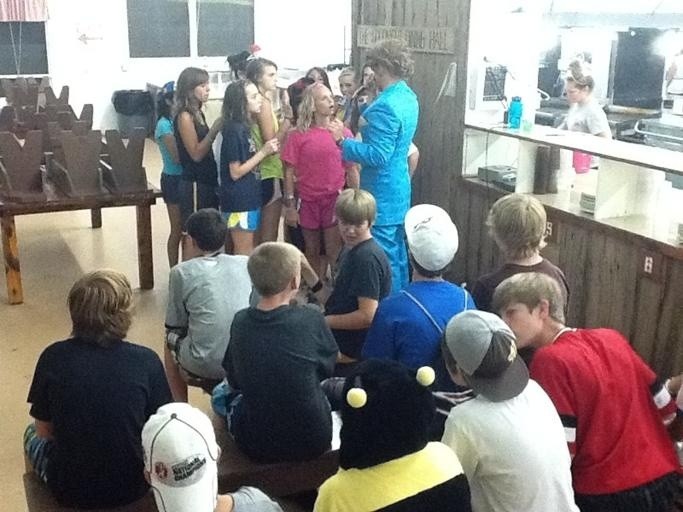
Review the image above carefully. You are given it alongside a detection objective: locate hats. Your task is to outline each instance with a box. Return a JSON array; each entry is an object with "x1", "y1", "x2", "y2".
[
  {"x1": 140, "y1": 401, "x2": 220, "y2": 512},
  {"x1": 444, "y1": 309, "x2": 530, "y2": 404},
  {"x1": 404, "y1": 203, "x2": 460, "y2": 272}
]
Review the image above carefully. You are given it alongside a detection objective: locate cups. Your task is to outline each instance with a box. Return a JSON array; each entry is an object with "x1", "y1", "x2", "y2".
[
  {"x1": 573, "y1": 151, "x2": 591, "y2": 174},
  {"x1": 578, "y1": 190, "x2": 595, "y2": 213}
]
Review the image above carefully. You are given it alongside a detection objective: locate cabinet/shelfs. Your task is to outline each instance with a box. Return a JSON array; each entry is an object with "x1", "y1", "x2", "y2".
[{"x1": 459, "y1": 124, "x2": 682, "y2": 261}]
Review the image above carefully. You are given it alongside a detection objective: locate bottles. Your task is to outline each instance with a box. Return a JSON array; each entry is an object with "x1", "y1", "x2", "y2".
[{"x1": 507, "y1": 97, "x2": 522, "y2": 128}]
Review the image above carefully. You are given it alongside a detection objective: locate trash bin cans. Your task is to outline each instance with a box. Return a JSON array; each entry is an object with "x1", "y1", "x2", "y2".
[{"x1": 111, "y1": 90, "x2": 154, "y2": 138}]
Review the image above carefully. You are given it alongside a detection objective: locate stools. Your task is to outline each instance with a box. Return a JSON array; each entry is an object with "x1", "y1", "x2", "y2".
[{"x1": 0, "y1": 74, "x2": 151, "y2": 199}]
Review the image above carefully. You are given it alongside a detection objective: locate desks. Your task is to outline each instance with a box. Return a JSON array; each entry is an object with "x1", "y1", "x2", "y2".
[{"x1": 0, "y1": 175, "x2": 163, "y2": 306}]
